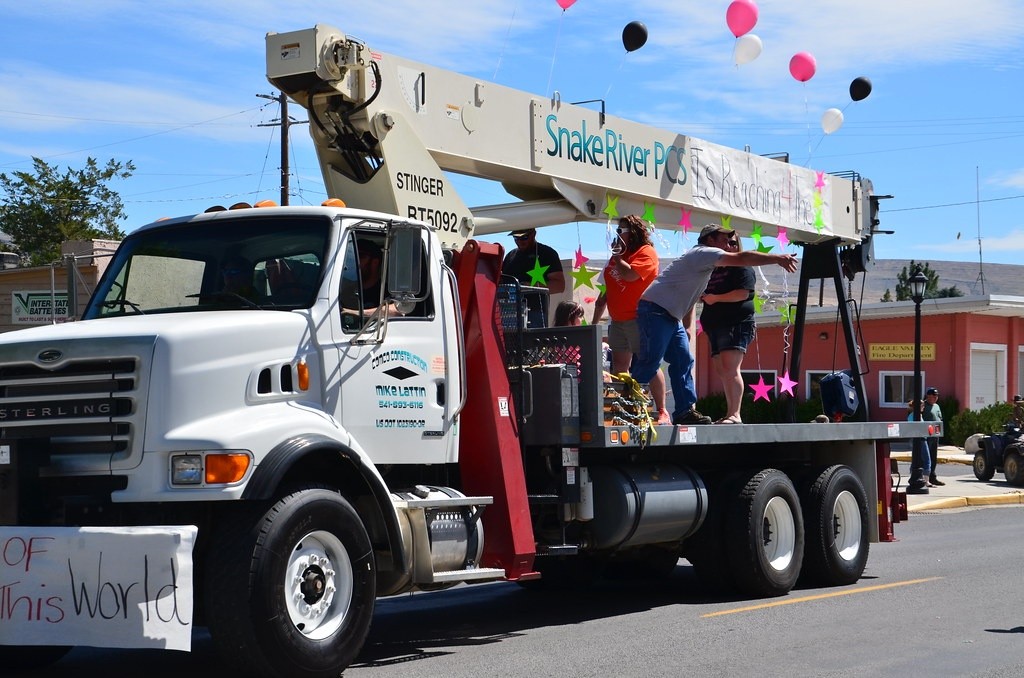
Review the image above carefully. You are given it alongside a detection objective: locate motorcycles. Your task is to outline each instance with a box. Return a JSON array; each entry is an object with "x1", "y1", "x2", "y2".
[{"x1": 972, "y1": 424, "x2": 1024, "y2": 487}]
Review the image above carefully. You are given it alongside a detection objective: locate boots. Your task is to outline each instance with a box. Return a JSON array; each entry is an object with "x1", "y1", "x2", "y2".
[{"x1": 924, "y1": 475, "x2": 932, "y2": 487}]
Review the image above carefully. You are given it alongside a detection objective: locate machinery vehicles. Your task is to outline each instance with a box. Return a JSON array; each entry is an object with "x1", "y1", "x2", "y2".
[{"x1": 0, "y1": 24, "x2": 945, "y2": 678}]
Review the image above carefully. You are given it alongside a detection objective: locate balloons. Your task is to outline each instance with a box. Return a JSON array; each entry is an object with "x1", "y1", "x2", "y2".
[
  {"x1": 735, "y1": 34, "x2": 762, "y2": 64},
  {"x1": 556, "y1": 0, "x2": 576, "y2": 9},
  {"x1": 726, "y1": 0, "x2": 759, "y2": 38},
  {"x1": 850, "y1": 77, "x2": 872, "y2": 100},
  {"x1": 789, "y1": 52, "x2": 816, "y2": 81},
  {"x1": 622, "y1": 21, "x2": 648, "y2": 52},
  {"x1": 823, "y1": 109, "x2": 844, "y2": 134}
]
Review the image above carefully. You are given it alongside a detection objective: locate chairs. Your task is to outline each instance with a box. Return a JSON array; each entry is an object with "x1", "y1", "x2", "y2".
[{"x1": 212, "y1": 257, "x2": 267, "y2": 307}]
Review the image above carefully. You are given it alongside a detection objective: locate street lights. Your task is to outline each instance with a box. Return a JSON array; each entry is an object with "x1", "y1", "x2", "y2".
[{"x1": 904, "y1": 263, "x2": 929, "y2": 495}]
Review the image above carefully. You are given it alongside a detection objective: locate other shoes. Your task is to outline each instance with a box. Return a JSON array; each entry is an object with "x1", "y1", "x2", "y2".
[
  {"x1": 657, "y1": 408, "x2": 670, "y2": 424},
  {"x1": 930, "y1": 479, "x2": 946, "y2": 486}
]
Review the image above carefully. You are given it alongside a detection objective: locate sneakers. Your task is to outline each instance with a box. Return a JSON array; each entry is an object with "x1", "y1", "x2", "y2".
[{"x1": 672, "y1": 409, "x2": 712, "y2": 424}]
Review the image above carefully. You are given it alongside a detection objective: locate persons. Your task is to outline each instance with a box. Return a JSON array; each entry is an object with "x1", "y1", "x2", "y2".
[
  {"x1": 554, "y1": 302, "x2": 584, "y2": 390},
  {"x1": 502, "y1": 228, "x2": 566, "y2": 363},
  {"x1": 632, "y1": 223, "x2": 798, "y2": 424},
  {"x1": 922, "y1": 388, "x2": 945, "y2": 485},
  {"x1": 908, "y1": 400, "x2": 933, "y2": 487},
  {"x1": 699, "y1": 232, "x2": 757, "y2": 423},
  {"x1": 341, "y1": 239, "x2": 405, "y2": 317},
  {"x1": 590, "y1": 215, "x2": 671, "y2": 425}
]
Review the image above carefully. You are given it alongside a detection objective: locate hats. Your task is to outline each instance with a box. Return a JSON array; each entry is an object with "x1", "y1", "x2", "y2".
[
  {"x1": 700, "y1": 223, "x2": 736, "y2": 239},
  {"x1": 927, "y1": 388, "x2": 939, "y2": 395},
  {"x1": 508, "y1": 229, "x2": 532, "y2": 237}
]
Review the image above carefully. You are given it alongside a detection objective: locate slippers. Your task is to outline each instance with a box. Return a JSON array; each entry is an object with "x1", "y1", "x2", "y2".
[{"x1": 715, "y1": 417, "x2": 743, "y2": 424}]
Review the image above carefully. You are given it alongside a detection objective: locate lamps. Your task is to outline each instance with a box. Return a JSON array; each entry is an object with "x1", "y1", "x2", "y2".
[{"x1": 818, "y1": 332, "x2": 828, "y2": 339}]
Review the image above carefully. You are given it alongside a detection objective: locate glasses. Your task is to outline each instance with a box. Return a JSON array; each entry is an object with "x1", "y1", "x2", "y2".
[
  {"x1": 513, "y1": 231, "x2": 532, "y2": 241},
  {"x1": 616, "y1": 227, "x2": 629, "y2": 235},
  {"x1": 728, "y1": 240, "x2": 738, "y2": 246}
]
